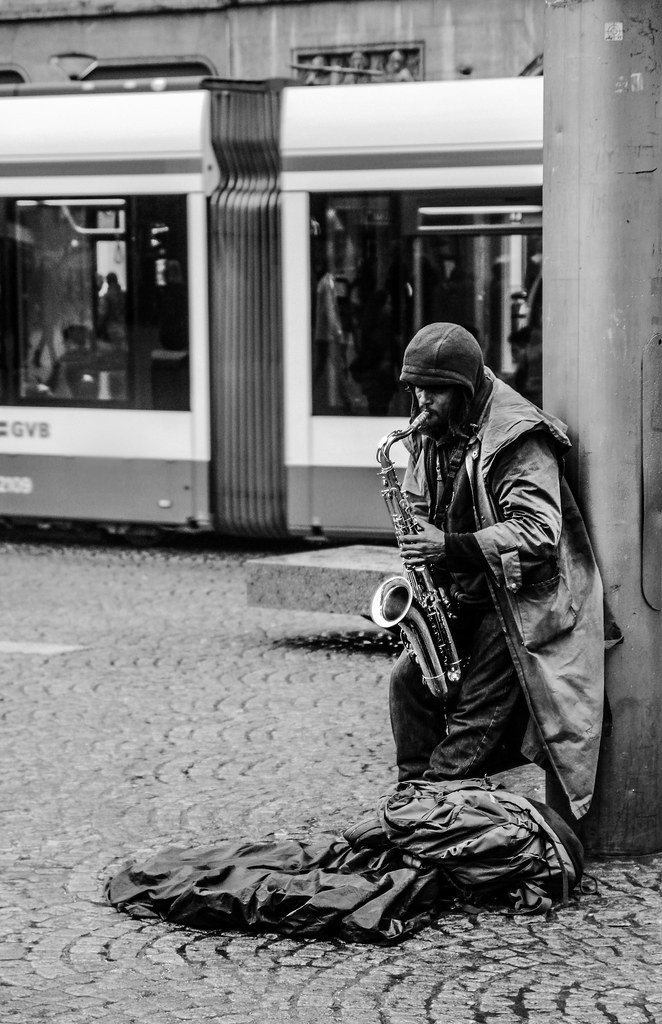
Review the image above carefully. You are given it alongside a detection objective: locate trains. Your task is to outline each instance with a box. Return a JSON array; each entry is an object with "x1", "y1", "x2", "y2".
[{"x1": 0, "y1": 73, "x2": 546, "y2": 547}]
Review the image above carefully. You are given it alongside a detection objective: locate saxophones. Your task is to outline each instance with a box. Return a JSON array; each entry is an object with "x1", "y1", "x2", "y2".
[{"x1": 366, "y1": 412, "x2": 472, "y2": 698}]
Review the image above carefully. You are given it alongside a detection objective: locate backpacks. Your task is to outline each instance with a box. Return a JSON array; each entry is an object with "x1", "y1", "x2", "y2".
[{"x1": 344, "y1": 778, "x2": 571, "y2": 915}]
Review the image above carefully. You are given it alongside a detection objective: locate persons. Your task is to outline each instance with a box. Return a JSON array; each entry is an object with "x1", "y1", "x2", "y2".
[
  {"x1": 44, "y1": 268, "x2": 190, "y2": 396},
  {"x1": 386, "y1": 320, "x2": 608, "y2": 820}
]
